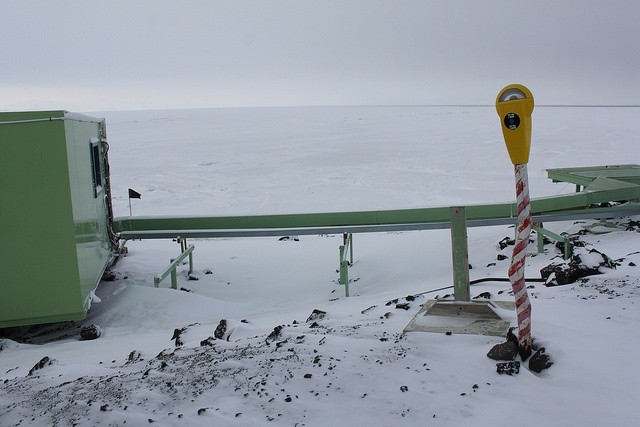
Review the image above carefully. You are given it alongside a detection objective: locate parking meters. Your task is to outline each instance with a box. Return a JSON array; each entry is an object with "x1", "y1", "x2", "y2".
[{"x1": 496, "y1": 83, "x2": 537, "y2": 354}]
